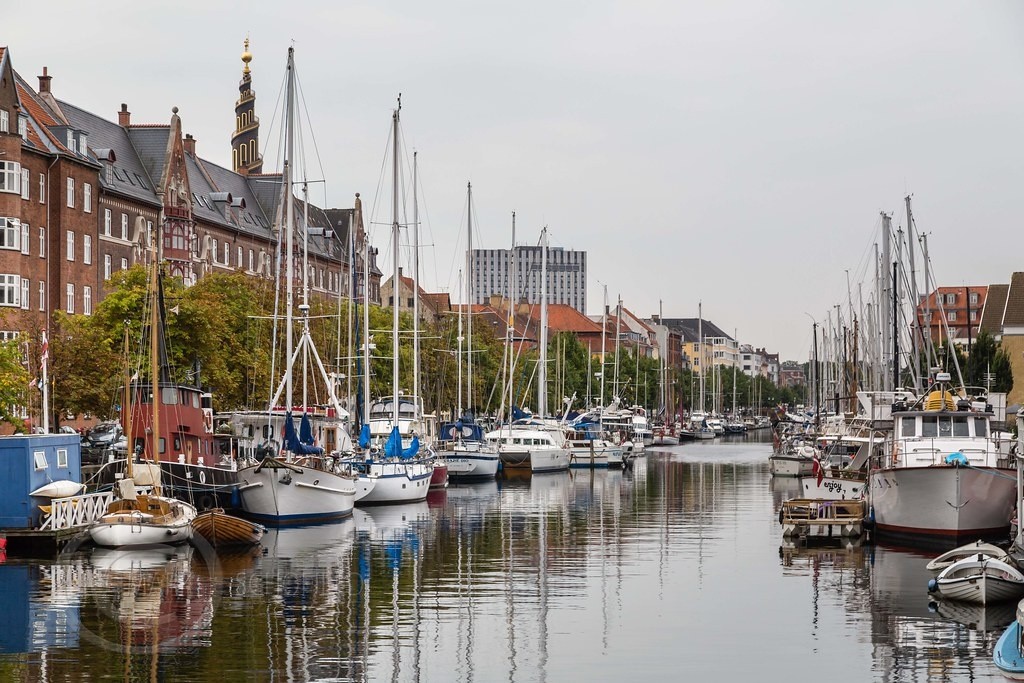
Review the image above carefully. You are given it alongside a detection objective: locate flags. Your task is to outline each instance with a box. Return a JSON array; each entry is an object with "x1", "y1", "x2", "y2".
[
  {"x1": 812, "y1": 453, "x2": 824, "y2": 488},
  {"x1": 772, "y1": 429, "x2": 781, "y2": 452},
  {"x1": 37, "y1": 336, "x2": 49, "y2": 369}
]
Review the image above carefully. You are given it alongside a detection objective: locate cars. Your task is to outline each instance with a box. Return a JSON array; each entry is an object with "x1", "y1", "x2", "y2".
[{"x1": 87, "y1": 423, "x2": 123, "y2": 449}]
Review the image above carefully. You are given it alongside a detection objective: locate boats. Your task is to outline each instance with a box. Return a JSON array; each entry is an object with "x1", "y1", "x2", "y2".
[
  {"x1": 927, "y1": 591, "x2": 1019, "y2": 642},
  {"x1": 992, "y1": 599, "x2": 1024, "y2": 681},
  {"x1": 925, "y1": 539, "x2": 1010, "y2": 578},
  {"x1": 937, "y1": 552, "x2": 1024, "y2": 606}
]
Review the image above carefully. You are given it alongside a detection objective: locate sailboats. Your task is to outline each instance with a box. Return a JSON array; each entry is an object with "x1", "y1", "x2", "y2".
[{"x1": 0, "y1": 39, "x2": 1023, "y2": 552}]
